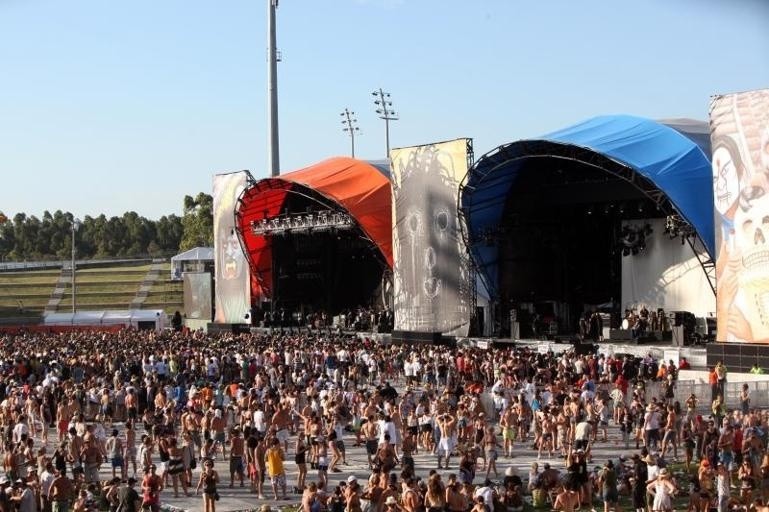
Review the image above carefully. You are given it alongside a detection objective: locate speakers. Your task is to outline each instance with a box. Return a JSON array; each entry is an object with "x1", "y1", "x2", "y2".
[
  {"x1": 672, "y1": 326, "x2": 688, "y2": 347},
  {"x1": 670, "y1": 311, "x2": 682, "y2": 326},
  {"x1": 510, "y1": 322, "x2": 527, "y2": 339},
  {"x1": 510, "y1": 308, "x2": 522, "y2": 322}
]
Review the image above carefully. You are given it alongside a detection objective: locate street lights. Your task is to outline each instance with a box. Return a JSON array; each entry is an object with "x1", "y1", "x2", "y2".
[
  {"x1": 340, "y1": 106, "x2": 362, "y2": 160},
  {"x1": 372, "y1": 87, "x2": 397, "y2": 160}
]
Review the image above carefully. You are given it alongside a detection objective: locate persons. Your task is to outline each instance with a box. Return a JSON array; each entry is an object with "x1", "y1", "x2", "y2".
[{"x1": 0, "y1": 292, "x2": 769, "y2": 510}]
